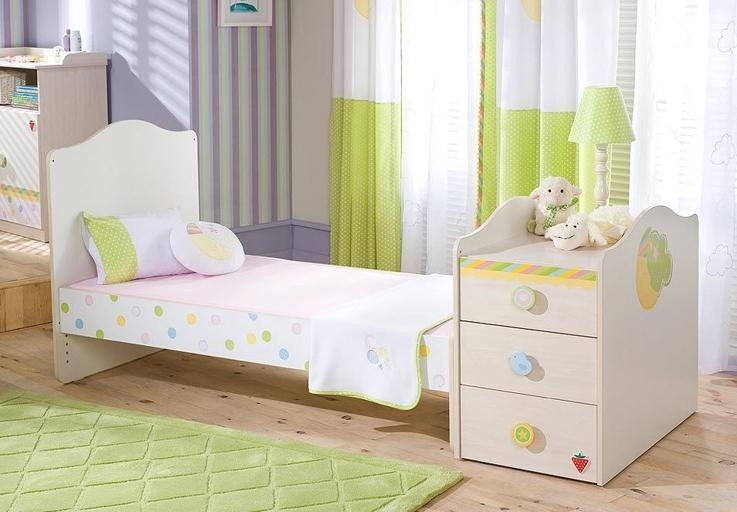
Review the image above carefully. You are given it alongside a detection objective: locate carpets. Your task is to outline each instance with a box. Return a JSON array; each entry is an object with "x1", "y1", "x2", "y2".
[{"x1": 1, "y1": 390, "x2": 468, "y2": 511}]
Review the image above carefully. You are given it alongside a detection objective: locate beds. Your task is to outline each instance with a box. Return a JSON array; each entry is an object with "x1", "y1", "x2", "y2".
[{"x1": 48, "y1": 120, "x2": 450, "y2": 393}]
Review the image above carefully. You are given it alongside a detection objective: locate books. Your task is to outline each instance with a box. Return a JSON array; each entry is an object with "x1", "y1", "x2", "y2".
[{"x1": 0, "y1": 69, "x2": 38, "y2": 112}]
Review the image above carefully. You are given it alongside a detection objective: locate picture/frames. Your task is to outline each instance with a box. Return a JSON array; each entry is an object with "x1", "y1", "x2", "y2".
[{"x1": 216, "y1": 1, "x2": 274, "y2": 27}]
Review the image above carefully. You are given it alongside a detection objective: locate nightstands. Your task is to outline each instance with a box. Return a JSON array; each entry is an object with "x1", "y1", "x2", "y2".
[{"x1": 451, "y1": 198, "x2": 700, "y2": 488}]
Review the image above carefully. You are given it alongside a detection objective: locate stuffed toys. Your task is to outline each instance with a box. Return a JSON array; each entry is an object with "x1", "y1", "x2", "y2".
[
  {"x1": 526, "y1": 176, "x2": 584, "y2": 237},
  {"x1": 541, "y1": 211, "x2": 624, "y2": 252}
]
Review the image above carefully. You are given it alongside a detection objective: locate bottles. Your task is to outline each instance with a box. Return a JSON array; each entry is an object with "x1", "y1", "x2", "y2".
[
  {"x1": 70, "y1": 30, "x2": 81, "y2": 52},
  {"x1": 61, "y1": 28, "x2": 70, "y2": 51}
]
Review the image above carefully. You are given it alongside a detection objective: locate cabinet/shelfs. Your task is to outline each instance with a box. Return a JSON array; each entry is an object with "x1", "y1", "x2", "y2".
[{"x1": 0, "y1": 44, "x2": 111, "y2": 242}]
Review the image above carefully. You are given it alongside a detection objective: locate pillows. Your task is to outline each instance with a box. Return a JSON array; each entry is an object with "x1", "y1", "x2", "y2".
[{"x1": 79, "y1": 207, "x2": 246, "y2": 286}]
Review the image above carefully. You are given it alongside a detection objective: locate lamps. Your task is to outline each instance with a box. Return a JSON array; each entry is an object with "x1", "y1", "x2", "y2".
[{"x1": 566, "y1": 86, "x2": 638, "y2": 210}]
